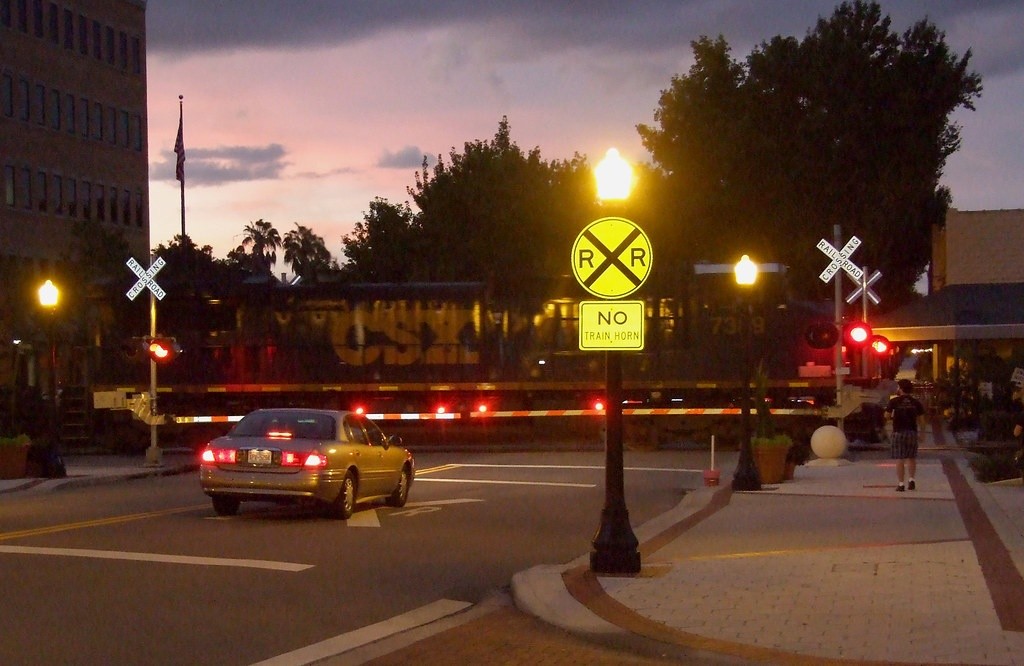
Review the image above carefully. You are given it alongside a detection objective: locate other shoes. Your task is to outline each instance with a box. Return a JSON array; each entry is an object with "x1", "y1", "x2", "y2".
[
  {"x1": 908, "y1": 481, "x2": 915, "y2": 489},
  {"x1": 896, "y1": 485, "x2": 904, "y2": 491}
]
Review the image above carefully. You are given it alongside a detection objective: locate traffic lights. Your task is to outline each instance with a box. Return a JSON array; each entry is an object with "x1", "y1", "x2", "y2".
[
  {"x1": 806, "y1": 322, "x2": 839, "y2": 349},
  {"x1": 868, "y1": 334, "x2": 891, "y2": 357},
  {"x1": 146, "y1": 337, "x2": 181, "y2": 365},
  {"x1": 120, "y1": 337, "x2": 149, "y2": 364},
  {"x1": 843, "y1": 321, "x2": 873, "y2": 348}
]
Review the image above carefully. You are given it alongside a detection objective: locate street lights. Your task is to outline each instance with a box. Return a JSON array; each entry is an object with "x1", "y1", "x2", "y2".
[
  {"x1": 588, "y1": 148, "x2": 640, "y2": 576},
  {"x1": 729, "y1": 255, "x2": 763, "y2": 490},
  {"x1": 38, "y1": 280, "x2": 70, "y2": 479}
]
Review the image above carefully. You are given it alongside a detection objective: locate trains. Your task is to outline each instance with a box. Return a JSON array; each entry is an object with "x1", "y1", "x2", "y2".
[{"x1": 94, "y1": 294, "x2": 850, "y2": 430}]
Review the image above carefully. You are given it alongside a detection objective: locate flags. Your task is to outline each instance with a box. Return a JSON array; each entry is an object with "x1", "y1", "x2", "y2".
[{"x1": 174, "y1": 105, "x2": 187, "y2": 183}]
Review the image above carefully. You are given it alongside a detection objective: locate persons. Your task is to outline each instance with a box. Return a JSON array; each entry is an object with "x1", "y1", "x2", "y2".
[{"x1": 884, "y1": 378, "x2": 927, "y2": 492}]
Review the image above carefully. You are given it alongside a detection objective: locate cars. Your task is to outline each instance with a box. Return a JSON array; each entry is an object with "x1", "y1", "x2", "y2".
[{"x1": 201, "y1": 408, "x2": 417, "y2": 519}]
